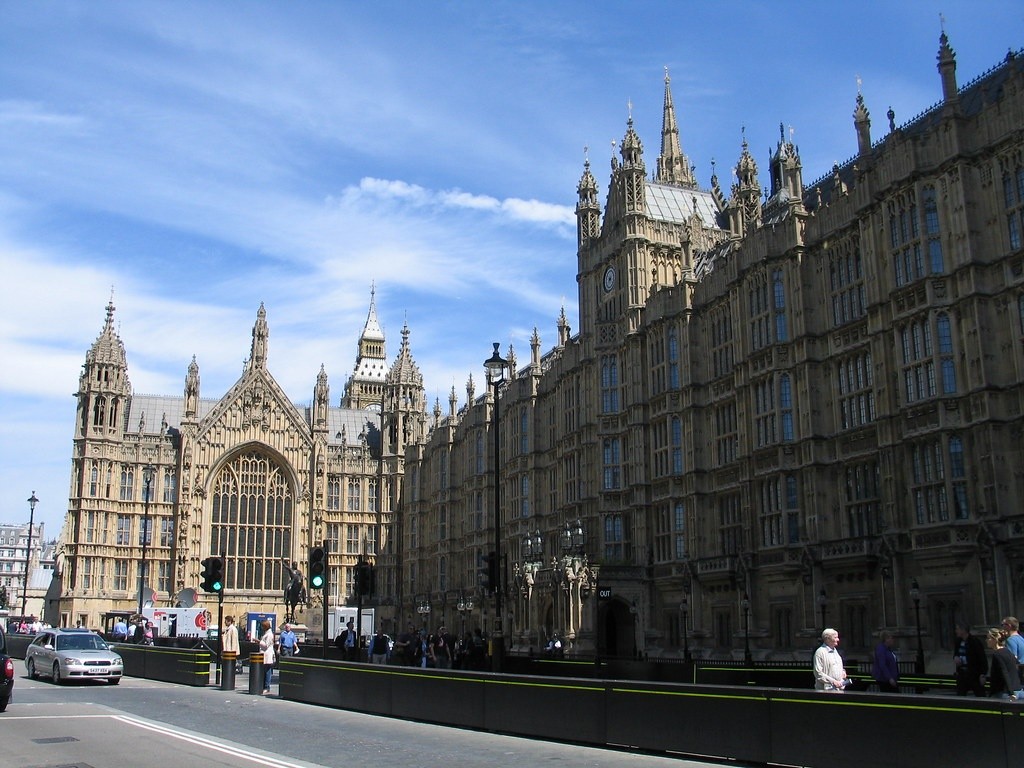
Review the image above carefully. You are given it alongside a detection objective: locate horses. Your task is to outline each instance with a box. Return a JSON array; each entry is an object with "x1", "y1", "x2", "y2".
[{"x1": 284, "y1": 571, "x2": 306, "y2": 623}]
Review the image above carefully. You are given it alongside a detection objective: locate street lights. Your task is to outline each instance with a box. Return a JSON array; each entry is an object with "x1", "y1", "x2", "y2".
[
  {"x1": 378, "y1": 610, "x2": 514, "y2": 650},
  {"x1": 457, "y1": 596, "x2": 473, "y2": 643},
  {"x1": 908, "y1": 581, "x2": 930, "y2": 690},
  {"x1": 17, "y1": 490, "x2": 39, "y2": 634},
  {"x1": 132, "y1": 458, "x2": 158, "y2": 645},
  {"x1": 417, "y1": 600, "x2": 430, "y2": 639},
  {"x1": 817, "y1": 585, "x2": 829, "y2": 631},
  {"x1": 680, "y1": 599, "x2": 688, "y2": 661},
  {"x1": 740, "y1": 596, "x2": 754, "y2": 667},
  {"x1": 481, "y1": 341, "x2": 512, "y2": 673}
]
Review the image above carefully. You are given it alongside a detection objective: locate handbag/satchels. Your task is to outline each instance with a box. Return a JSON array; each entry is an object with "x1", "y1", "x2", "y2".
[{"x1": 236, "y1": 659, "x2": 243, "y2": 675}]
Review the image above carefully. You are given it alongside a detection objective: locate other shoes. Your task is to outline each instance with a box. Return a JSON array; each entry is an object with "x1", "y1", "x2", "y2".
[{"x1": 263, "y1": 689, "x2": 270, "y2": 693}]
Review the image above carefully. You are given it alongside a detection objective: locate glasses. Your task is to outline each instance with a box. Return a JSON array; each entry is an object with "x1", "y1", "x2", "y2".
[
  {"x1": 439, "y1": 627, "x2": 445, "y2": 630},
  {"x1": 408, "y1": 628, "x2": 415, "y2": 630},
  {"x1": 1001, "y1": 624, "x2": 1013, "y2": 626}
]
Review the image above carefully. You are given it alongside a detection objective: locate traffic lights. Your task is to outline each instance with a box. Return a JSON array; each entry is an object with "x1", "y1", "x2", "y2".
[
  {"x1": 199, "y1": 558, "x2": 210, "y2": 592},
  {"x1": 309, "y1": 547, "x2": 326, "y2": 589},
  {"x1": 353, "y1": 563, "x2": 369, "y2": 594},
  {"x1": 481, "y1": 551, "x2": 495, "y2": 591},
  {"x1": 211, "y1": 557, "x2": 224, "y2": 593}
]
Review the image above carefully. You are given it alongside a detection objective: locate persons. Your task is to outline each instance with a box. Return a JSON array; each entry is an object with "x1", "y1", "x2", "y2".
[
  {"x1": 368, "y1": 629, "x2": 391, "y2": 665},
  {"x1": 5, "y1": 613, "x2": 62, "y2": 637},
  {"x1": 280, "y1": 556, "x2": 306, "y2": 606},
  {"x1": 985, "y1": 617, "x2": 1024, "y2": 702},
  {"x1": 71, "y1": 619, "x2": 85, "y2": 630},
  {"x1": 395, "y1": 624, "x2": 493, "y2": 670},
  {"x1": 168, "y1": 614, "x2": 177, "y2": 637},
  {"x1": 870, "y1": 630, "x2": 902, "y2": 693},
  {"x1": 111, "y1": 616, "x2": 154, "y2": 647},
  {"x1": 335, "y1": 621, "x2": 356, "y2": 661},
  {"x1": 223, "y1": 616, "x2": 240, "y2": 656},
  {"x1": 277, "y1": 624, "x2": 300, "y2": 657},
  {"x1": 253, "y1": 620, "x2": 276, "y2": 695},
  {"x1": 544, "y1": 634, "x2": 561, "y2": 652},
  {"x1": 811, "y1": 628, "x2": 853, "y2": 692},
  {"x1": 954, "y1": 619, "x2": 989, "y2": 697}
]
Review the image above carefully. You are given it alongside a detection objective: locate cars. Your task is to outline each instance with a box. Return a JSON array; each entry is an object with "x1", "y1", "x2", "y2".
[
  {"x1": 0, "y1": 624, "x2": 15, "y2": 714},
  {"x1": 24, "y1": 628, "x2": 125, "y2": 686}
]
